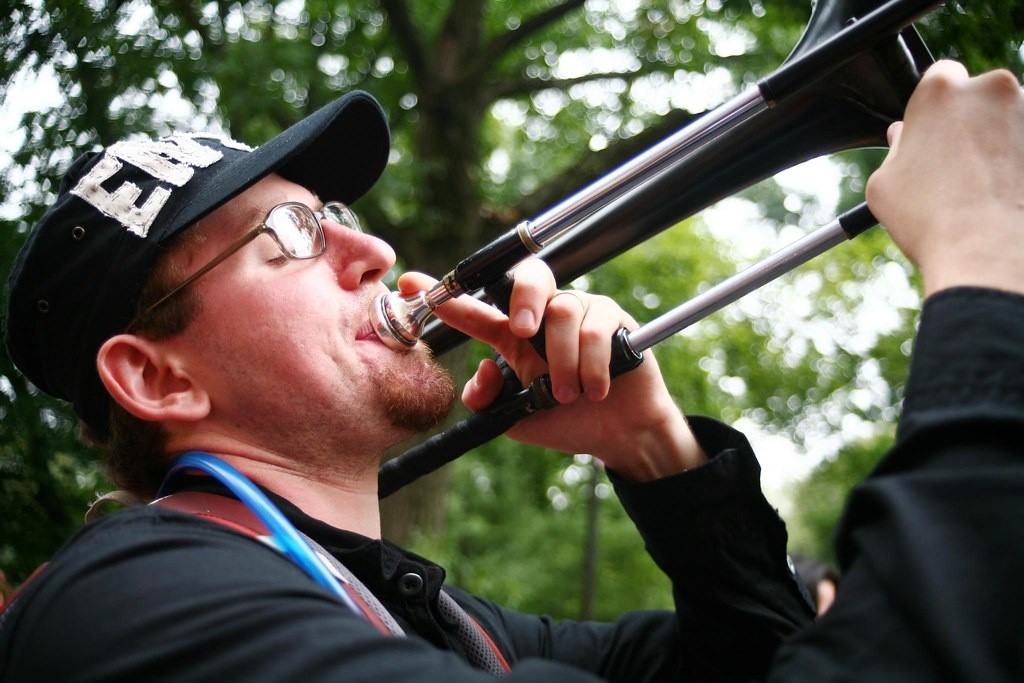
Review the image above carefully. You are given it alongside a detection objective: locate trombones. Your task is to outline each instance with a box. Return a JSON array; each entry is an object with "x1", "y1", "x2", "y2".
[{"x1": 378, "y1": 0, "x2": 1024, "y2": 499}]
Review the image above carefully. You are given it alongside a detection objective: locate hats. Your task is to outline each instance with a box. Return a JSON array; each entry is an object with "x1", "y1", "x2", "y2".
[{"x1": 4, "y1": 89, "x2": 390, "y2": 401}]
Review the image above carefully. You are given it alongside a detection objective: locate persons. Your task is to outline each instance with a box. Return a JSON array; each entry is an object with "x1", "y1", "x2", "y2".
[{"x1": 0, "y1": 59, "x2": 1024, "y2": 683}]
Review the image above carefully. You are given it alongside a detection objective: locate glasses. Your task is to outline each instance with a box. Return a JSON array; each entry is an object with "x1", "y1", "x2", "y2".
[{"x1": 122, "y1": 202, "x2": 364, "y2": 332}]
